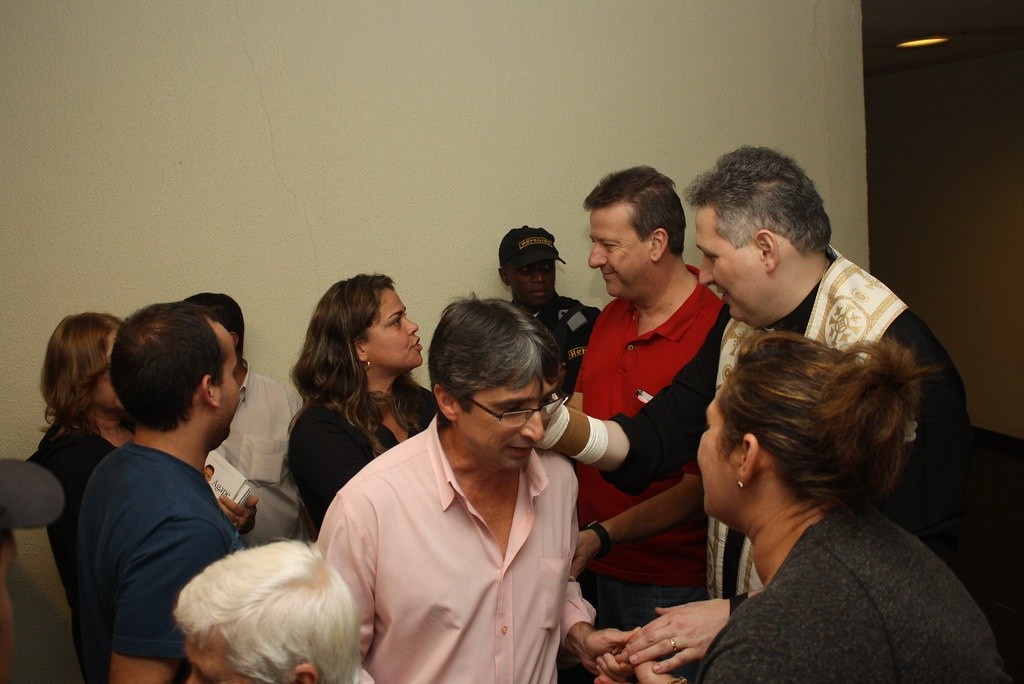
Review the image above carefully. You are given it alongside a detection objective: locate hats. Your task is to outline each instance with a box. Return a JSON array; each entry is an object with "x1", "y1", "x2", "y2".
[
  {"x1": 0, "y1": 457, "x2": 66, "y2": 528},
  {"x1": 498, "y1": 225, "x2": 566, "y2": 268}
]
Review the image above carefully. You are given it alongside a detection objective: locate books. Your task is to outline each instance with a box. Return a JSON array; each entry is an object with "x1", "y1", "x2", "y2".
[{"x1": 204, "y1": 450, "x2": 251, "y2": 507}]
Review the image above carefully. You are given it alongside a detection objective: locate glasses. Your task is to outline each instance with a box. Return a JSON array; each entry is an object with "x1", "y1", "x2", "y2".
[{"x1": 465, "y1": 388, "x2": 572, "y2": 428}]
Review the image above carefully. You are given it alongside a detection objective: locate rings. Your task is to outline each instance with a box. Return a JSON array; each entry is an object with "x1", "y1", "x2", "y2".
[
  {"x1": 236, "y1": 516, "x2": 238, "y2": 522},
  {"x1": 669, "y1": 638, "x2": 676, "y2": 652}
]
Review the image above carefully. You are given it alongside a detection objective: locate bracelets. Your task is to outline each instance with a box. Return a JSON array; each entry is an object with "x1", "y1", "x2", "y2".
[
  {"x1": 580, "y1": 520, "x2": 612, "y2": 560},
  {"x1": 667, "y1": 676, "x2": 688, "y2": 684}
]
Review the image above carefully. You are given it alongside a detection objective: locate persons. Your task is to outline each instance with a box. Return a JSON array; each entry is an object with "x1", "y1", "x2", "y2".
[
  {"x1": 288, "y1": 273, "x2": 437, "y2": 535},
  {"x1": 316, "y1": 300, "x2": 641, "y2": 684},
  {"x1": 204, "y1": 465, "x2": 214, "y2": 482},
  {"x1": 629, "y1": 330, "x2": 1010, "y2": 684},
  {"x1": 24, "y1": 312, "x2": 259, "y2": 684},
  {"x1": 173, "y1": 540, "x2": 375, "y2": 684},
  {"x1": 566, "y1": 165, "x2": 725, "y2": 630},
  {"x1": 0, "y1": 456, "x2": 64, "y2": 684},
  {"x1": 72, "y1": 301, "x2": 245, "y2": 684},
  {"x1": 537, "y1": 146, "x2": 974, "y2": 674},
  {"x1": 184, "y1": 293, "x2": 318, "y2": 549},
  {"x1": 499, "y1": 226, "x2": 600, "y2": 396}
]
